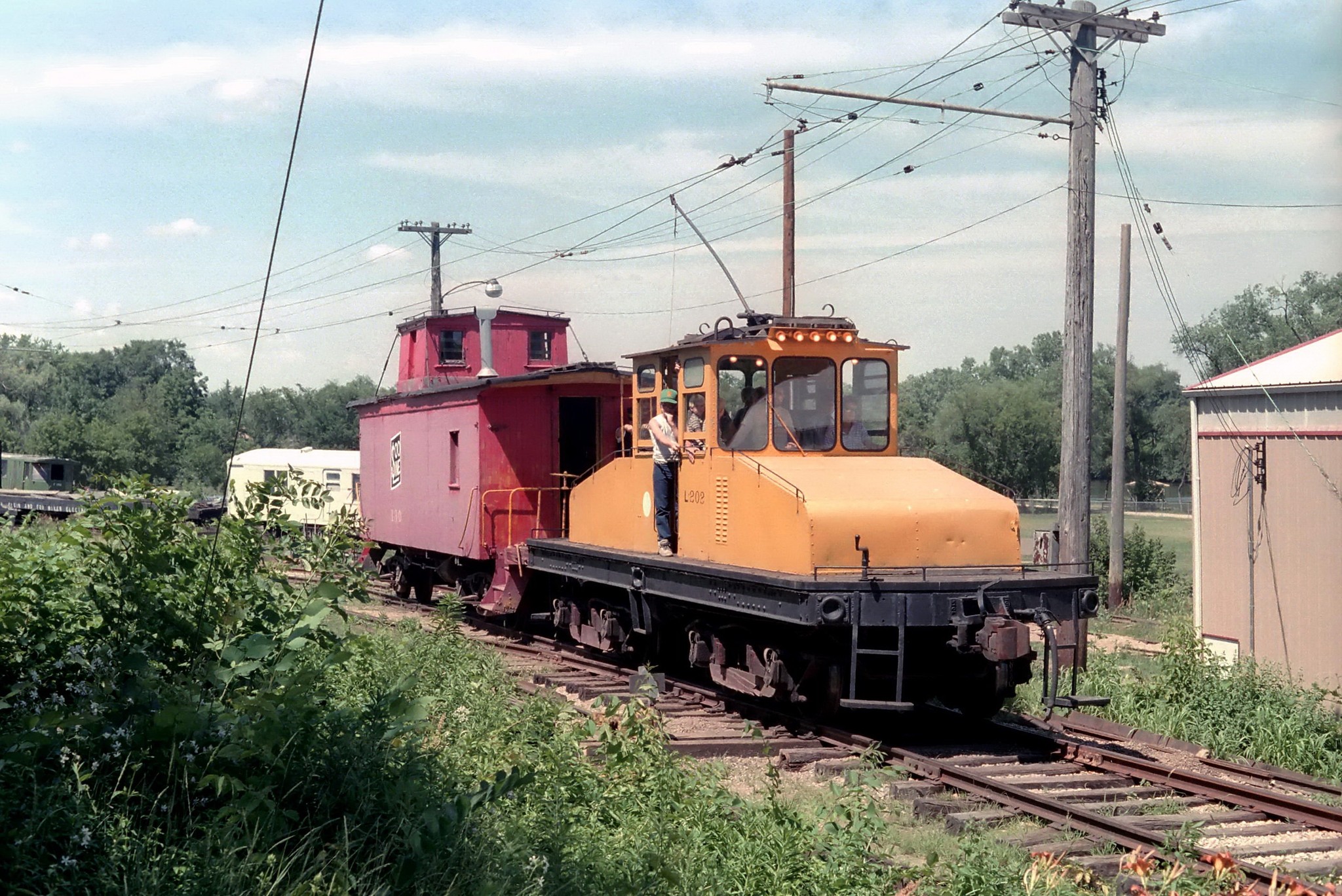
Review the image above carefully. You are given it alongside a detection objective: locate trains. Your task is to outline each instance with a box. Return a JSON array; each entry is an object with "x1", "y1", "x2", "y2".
[{"x1": 341, "y1": 128, "x2": 1107, "y2": 734}]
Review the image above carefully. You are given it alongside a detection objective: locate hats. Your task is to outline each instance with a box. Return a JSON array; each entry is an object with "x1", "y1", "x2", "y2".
[
  {"x1": 627, "y1": 407, "x2": 632, "y2": 413},
  {"x1": 661, "y1": 389, "x2": 678, "y2": 404},
  {"x1": 752, "y1": 371, "x2": 767, "y2": 389}
]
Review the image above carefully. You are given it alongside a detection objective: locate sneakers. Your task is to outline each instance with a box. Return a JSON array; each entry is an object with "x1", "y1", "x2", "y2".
[{"x1": 658, "y1": 539, "x2": 673, "y2": 557}]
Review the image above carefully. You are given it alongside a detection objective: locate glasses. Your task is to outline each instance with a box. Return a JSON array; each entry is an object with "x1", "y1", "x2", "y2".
[
  {"x1": 628, "y1": 417, "x2": 632, "y2": 421},
  {"x1": 661, "y1": 404, "x2": 676, "y2": 409}
]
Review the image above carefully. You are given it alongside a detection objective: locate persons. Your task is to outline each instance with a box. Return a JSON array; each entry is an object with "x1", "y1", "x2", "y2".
[
  {"x1": 615, "y1": 383, "x2": 835, "y2": 557},
  {"x1": 824, "y1": 396, "x2": 886, "y2": 452}
]
